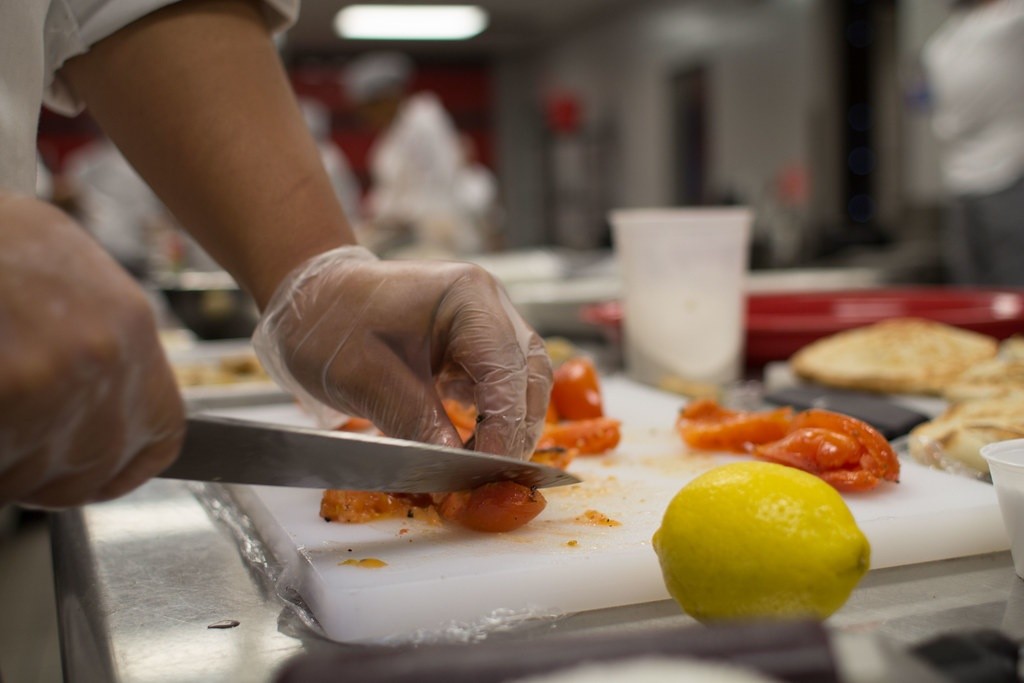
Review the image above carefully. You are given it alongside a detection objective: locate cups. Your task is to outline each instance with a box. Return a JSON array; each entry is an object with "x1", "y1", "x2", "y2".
[
  {"x1": 980, "y1": 436, "x2": 1024, "y2": 579},
  {"x1": 608, "y1": 205, "x2": 749, "y2": 397}
]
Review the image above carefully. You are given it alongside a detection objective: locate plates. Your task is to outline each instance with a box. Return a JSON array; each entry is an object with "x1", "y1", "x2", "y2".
[{"x1": 582, "y1": 286, "x2": 1024, "y2": 367}]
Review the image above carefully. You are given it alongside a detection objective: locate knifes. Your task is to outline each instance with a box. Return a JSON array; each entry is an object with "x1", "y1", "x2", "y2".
[{"x1": 151, "y1": 413, "x2": 580, "y2": 492}]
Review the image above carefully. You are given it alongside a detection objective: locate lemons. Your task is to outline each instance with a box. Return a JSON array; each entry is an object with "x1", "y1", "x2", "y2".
[{"x1": 649, "y1": 461, "x2": 870, "y2": 624}]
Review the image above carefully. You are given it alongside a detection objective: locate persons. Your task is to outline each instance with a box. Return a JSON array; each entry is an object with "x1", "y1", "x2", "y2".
[
  {"x1": 920, "y1": 0, "x2": 1024, "y2": 288},
  {"x1": 1, "y1": 0, "x2": 557, "y2": 683},
  {"x1": 449, "y1": 127, "x2": 502, "y2": 252},
  {"x1": 299, "y1": 97, "x2": 363, "y2": 221},
  {"x1": 55, "y1": 133, "x2": 168, "y2": 282}
]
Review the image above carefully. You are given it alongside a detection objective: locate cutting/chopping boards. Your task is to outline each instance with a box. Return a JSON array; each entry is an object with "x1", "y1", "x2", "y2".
[{"x1": 195, "y1": 374, "x2": 1006, "y2": 646}]
